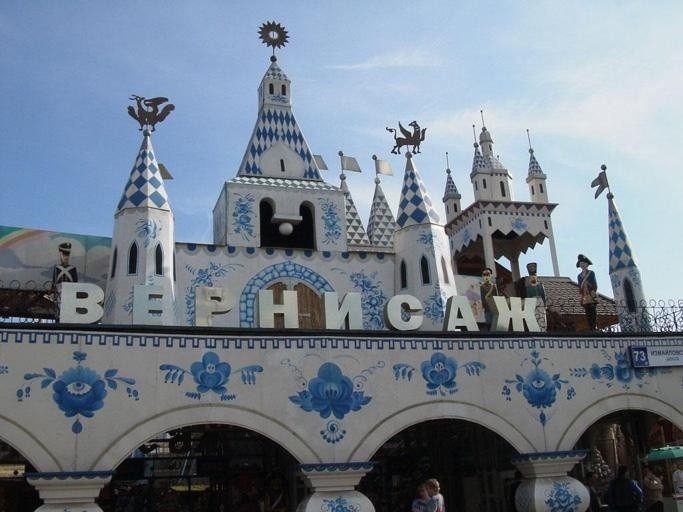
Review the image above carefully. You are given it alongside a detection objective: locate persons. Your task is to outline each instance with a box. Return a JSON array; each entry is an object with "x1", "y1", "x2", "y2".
[
  {"x1": 424, "y1": 478, "x2": 445, "y2": 511},
  {"x1": 53, "y1": 249, "x2": 76, "y2": 326},
  {"x1": 526, "y1": 273, "x2": 547, "y2": 331},
  {"x1": 479, "y1": 268, "x2": 499, "y2": 333},
  {"x1": 576, "y1": 254, "x2": 598, "y2": 329},
  {"x1": 412, "y1": 484, "x2": 430, "y2": 512},
  {"x1": 672, "y1": 462, "x2": 683, "y2": 493},
  {"x1": 640, "y1": 465, "x2": 665, "y2": 512},
  {"x1": 606, "y1": 463, "x2": 644, "y2": 512}
]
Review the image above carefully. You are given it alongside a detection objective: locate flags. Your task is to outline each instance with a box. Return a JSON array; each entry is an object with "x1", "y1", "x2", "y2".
[
  {"x1": 313, "y1": 154, "x2": 327, "y2": 171},
  {"x1": 374, "y1": 158, "x2": 394, "y2": 177},
  {"x1": 339, "y1": 154, "x2": 362, "y2": 173}
]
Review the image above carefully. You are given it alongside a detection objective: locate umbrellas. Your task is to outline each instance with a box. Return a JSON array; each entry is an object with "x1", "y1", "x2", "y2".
[{"x1": 643, "y1": 443, "x2": 683, "y2": 482}]
